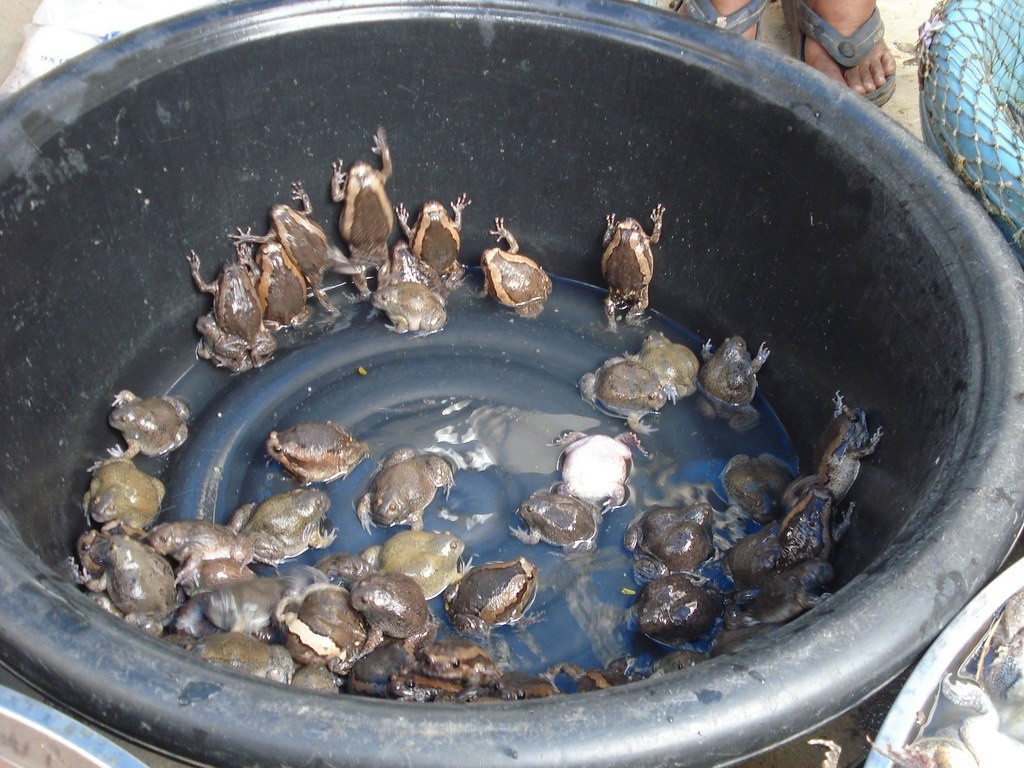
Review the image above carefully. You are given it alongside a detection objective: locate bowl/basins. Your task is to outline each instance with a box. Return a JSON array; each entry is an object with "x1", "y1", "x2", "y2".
[
  {"x1": 0, "y1": 684, "x2": 151, "y2": 768},
  {"x1": 0, "y1": 0, "x2": 1024, "y2": 768},
  {"x1": 862, "y1": 557, "x2": 1024, "y2": 768}
]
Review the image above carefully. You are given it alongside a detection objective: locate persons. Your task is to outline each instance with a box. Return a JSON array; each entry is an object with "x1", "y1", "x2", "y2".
[{"x1": 671, "y1": 0, "x2": 896, "y2": 110}]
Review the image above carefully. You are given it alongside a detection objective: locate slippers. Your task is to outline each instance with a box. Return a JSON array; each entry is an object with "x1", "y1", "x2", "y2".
[
  {"x1": 669, "y1": 0, "x2": 771, "y2": 42},
  {"x1": 781, "y1": 0, "x2": 896, "y2": 108}
]
